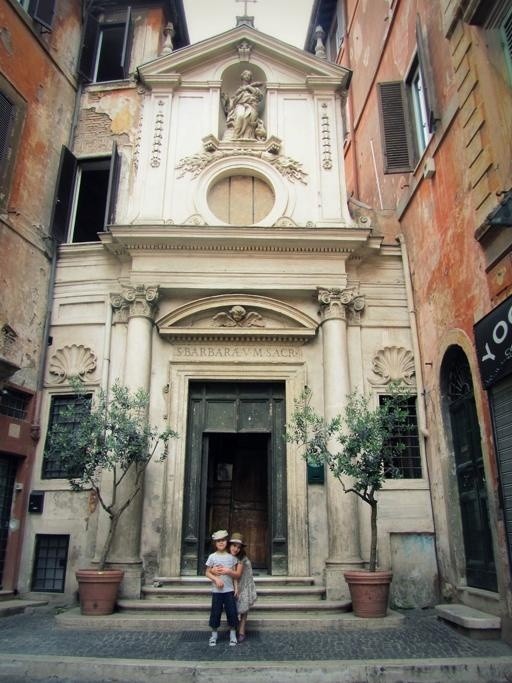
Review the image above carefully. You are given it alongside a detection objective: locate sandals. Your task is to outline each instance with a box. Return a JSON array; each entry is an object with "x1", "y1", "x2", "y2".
[{"x1": 238, "y1": 632, "x2": 246, "y2": 643}]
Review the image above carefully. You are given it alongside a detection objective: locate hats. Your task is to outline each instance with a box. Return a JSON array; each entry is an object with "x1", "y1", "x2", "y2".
[
  {"x1": 227, "y1": 532, "x2": 248, "y2": 548},
  {"x1": 211, "y1": 529, "x2": 229, "y2": 540}
]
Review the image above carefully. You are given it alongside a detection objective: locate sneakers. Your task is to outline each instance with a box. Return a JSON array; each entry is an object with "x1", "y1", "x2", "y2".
[
  {"x1": 208, "y1": 637, "x2": 217, "y2": 647},
  {"x1": 229, "y1": 639, "x2": 238, "y2": 647}
]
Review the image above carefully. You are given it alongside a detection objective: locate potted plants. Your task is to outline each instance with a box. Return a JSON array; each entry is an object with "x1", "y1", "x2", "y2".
[
  {"x1": 47, "y1": 368, "x2": 182, "y2": 617},
  {"x1": 282, "y1": 377, "x2": 414, "y2": 618}
]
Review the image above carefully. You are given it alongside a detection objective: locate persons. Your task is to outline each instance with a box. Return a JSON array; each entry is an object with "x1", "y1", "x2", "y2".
[
  {"x1": 229, "y1": 304, "x2": 246, "y2": 322},
  {"x1": 204, "y1": 529, "x2": 240, "y2": 648},
  {"x1": 211, "y1": 532, "x2": 258, "y2": 643},
  {"x1": 230, "y1": 69, "x2": 264, "y2": 142}
]
